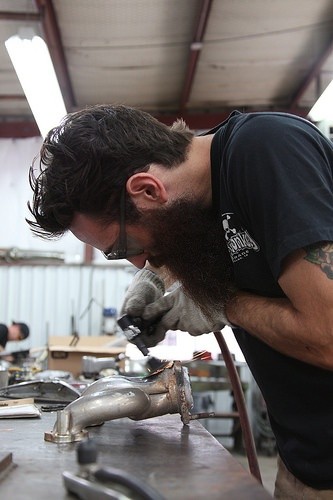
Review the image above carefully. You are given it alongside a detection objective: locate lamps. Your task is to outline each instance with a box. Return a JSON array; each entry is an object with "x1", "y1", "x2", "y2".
[{"x1": 4, "y1": 26, "x2": 68, "y2": 143}]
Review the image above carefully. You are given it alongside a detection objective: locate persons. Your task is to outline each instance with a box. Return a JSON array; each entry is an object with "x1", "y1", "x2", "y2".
[
  {"x1": 24, "y1": 102, "x2": 333, "y2": 500},
  {"x1": 0, "y1": 319, "x2": 29, "y2": 352}
]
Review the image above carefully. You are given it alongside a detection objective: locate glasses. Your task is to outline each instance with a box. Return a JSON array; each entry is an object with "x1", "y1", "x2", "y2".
[{"x1": 101, "y1": 164, "x2": 150, "y2": 260}]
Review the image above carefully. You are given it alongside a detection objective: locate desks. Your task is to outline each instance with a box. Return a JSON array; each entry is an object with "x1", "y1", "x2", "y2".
[
  {"x1": 180, "y1": 361, "x2": 250, "y2": 454},
  {"x1": 0, "y1": 375, "x2": 275, "y2": 500}
]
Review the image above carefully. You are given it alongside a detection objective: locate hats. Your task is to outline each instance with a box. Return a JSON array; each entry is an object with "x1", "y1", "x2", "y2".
[{"x1": 12, "y1": 321, "x2": 28, "y2": 339}]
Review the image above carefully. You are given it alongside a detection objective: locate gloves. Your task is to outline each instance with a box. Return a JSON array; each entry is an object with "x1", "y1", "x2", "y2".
[{"x1": 119, "y1": 268, "x2": 240, "y2": 348}]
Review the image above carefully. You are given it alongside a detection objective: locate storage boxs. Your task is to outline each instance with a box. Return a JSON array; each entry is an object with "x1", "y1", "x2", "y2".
[{"x1": 46, "y1": 335, "x2": 127, "y2": 377}]
